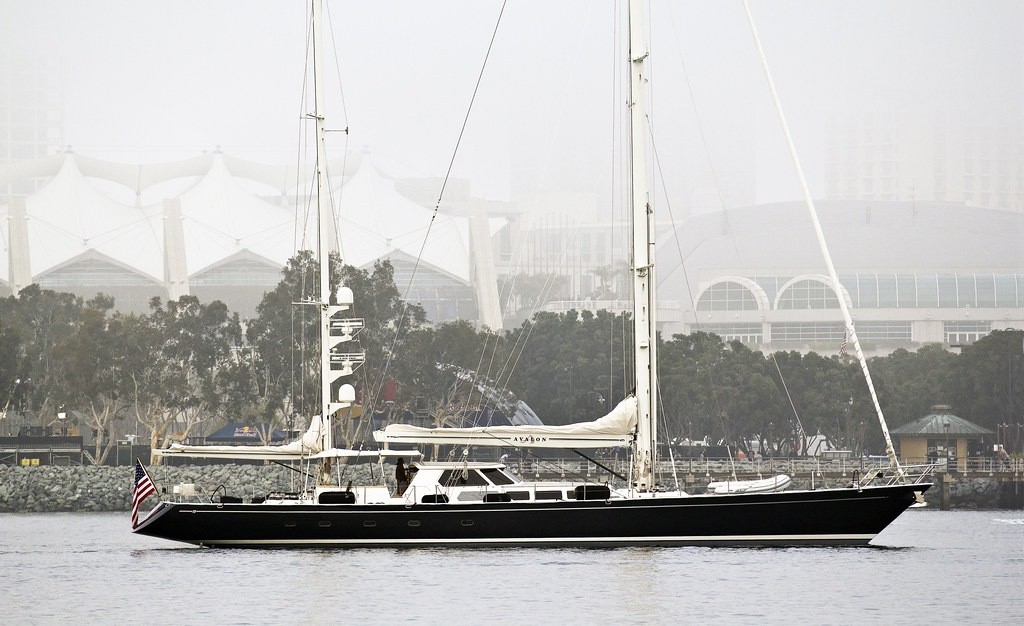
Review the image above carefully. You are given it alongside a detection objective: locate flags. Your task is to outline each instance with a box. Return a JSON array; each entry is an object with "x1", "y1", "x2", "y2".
[
  {"x1": 839, "y1": 331, "x2": 846, "y2": 356},
  {"x1": 131, "y1": 456, "x2": 156, "y2": 530}
]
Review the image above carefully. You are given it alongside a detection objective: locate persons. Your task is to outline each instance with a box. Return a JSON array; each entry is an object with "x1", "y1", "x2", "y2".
[
  {"x1": 595, "y1": 449, "x2": 602, "y2": 458},
  {"x1": 396, "y1": 457, "x2": 408, "y2": 481},
  {"x1": 699, "y1": 451, "x2": 705, "y2": 461},
  {"x1": 500, "y1": 454, "x2": 510, "y2": 464},
  {"x1": 999, "y1": 444, "x2": 1010, "y2": 468},
  {"x1": 754, "y1": 451, "x2": 762, "y2": 465},
  {"x1": 527, "y1": 449, "x2": 534, "y2": 462},
  {"x1": 674, "y1": 451, "x2": 683, "y2": 460},
  {"x1": 925, "y1": 448, "x2": 938, "y2": 464}
]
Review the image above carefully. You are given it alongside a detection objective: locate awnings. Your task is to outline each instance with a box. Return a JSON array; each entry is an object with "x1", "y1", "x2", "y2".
[{"x1": 205, "y1": 423, "x2": 286, "y2": 441}]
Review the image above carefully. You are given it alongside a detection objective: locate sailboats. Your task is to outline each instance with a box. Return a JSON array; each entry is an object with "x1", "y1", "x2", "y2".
[{"x1": 129, "y1": 1, "x2": 937, "y2": 550}]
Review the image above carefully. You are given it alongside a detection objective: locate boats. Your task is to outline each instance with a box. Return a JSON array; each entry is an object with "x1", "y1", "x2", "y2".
[{"x1": 707, "y1": 475, "x2": 791, "y2": 494}]
